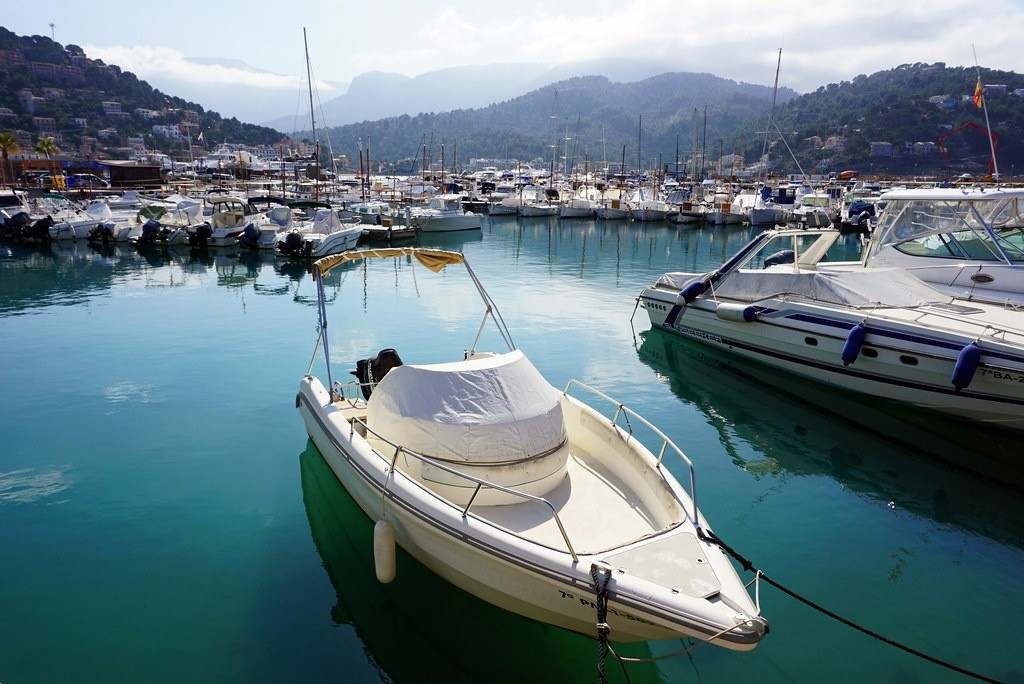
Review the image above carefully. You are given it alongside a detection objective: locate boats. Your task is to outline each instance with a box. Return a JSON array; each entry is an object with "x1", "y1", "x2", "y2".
[
  {"x1": 300, "y1": 438, "x2": 662, "y2": 684},
  {"x1": 641, "y1": 116, "x2": 1024, "y2": 429},
  {"x1": 0, "y1": 28, "x2": 1024, "y2": 260},
  {"x1": 294, "y1": 247, "x2": 769, "y2": 651},
  {"x1": 817, "y1": 42, "x2": 1024, "y2": 305}
]
